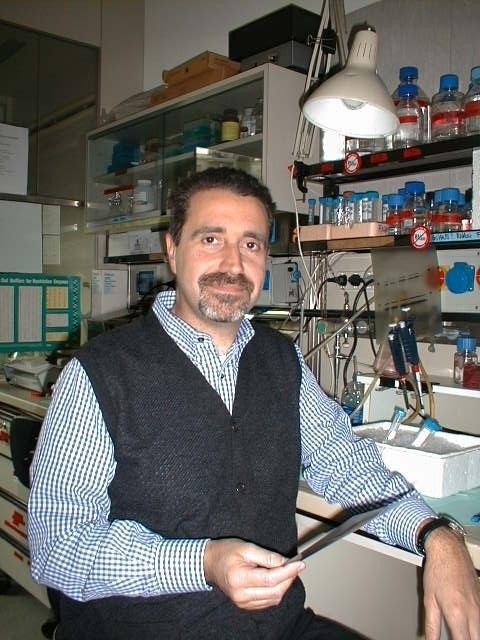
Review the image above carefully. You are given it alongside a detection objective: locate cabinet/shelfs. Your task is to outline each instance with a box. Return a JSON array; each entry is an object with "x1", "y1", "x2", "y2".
[
  {"x1": 290, "y1": 134, "x2": 480, "y2": 248},
  {"x1": 83, "y1": 61, "x2": 322, "y2": 236}
]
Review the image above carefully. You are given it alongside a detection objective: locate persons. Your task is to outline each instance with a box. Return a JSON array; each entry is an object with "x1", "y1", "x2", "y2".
[{"x1": 19, "y1": 165, "x2": 480, "y2": 640}]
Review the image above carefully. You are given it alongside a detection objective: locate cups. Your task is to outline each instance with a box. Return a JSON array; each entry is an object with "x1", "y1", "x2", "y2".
[{"x1": 410, "y1": 417, "x2": 441, "y2": 449}]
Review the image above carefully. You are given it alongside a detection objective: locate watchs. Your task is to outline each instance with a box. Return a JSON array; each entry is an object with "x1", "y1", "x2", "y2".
[{"x1": 414, "y1": 511, "x2": 465, "y2": 555}]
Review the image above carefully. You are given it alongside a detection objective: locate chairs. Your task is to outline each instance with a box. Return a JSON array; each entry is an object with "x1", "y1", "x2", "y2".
[{"x1": 8, "y1": 416, "x2": 62, "y2": 640}]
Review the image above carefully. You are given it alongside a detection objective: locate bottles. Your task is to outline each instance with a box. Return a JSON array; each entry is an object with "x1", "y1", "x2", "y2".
[
  {"x1": 371, "y1": 407, "x2": 407, "y2": 443},
  {"x1": 388, "y1": 321, "x2": 419, "y2": 375},
  {"x1": 385, "y1": 66, "x2": 480, "y2": 151},
  {"x1": 454, "y1": 332, "x2": 478, "y2": 385},
  {"x1": 309, "y1": 181, "x2": 472, "y2": 235},
  {"x1": 341, "y1": 370, "x2": 363, "y2": 426},
  {"x1": 133, "y1": 179, "x2": 154, "y2": 214},
  {"x1": 222, "y1": 96, "x2": 263, "y2": 143}
]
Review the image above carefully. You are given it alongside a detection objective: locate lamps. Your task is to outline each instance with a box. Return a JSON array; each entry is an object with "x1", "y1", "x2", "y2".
[{"x1": 290, "y1": 0, "x2": 402, "y2": 162}]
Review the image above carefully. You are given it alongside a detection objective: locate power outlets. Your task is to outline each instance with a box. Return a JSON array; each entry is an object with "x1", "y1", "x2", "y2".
[{"x1": 335, "y1": 271, "x2": 375, "y2": 290}]
